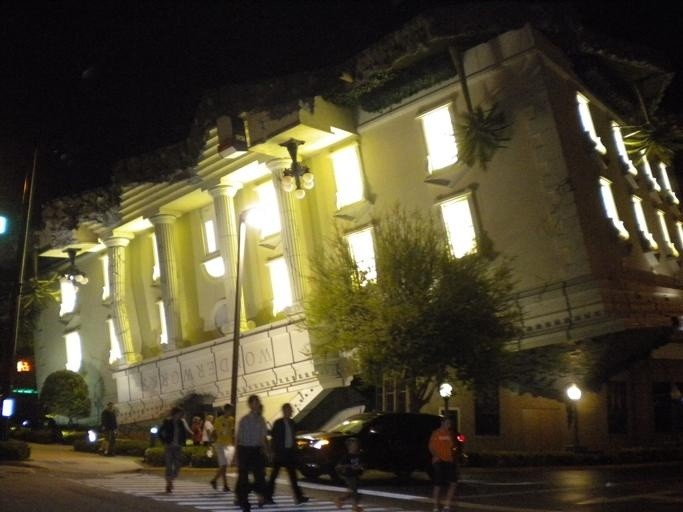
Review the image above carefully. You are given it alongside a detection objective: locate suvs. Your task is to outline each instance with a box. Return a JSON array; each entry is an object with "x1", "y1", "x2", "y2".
[{"x1": 293, "y1": 407, "x2": 468, "y2": 487}]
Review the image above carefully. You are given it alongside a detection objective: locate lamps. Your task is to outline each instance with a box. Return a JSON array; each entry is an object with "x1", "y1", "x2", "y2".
[
  {"x1": 62, "y1": 245, "x2": 90, "y2": 288},
  {"x1": 277, "y1": 135, "x2": 315, "y2": 201}
]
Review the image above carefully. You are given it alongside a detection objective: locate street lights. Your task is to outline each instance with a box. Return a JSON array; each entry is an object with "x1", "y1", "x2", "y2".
[
  {"x1": 225, "y1": 201, "x2": 268, "y2": 442},
  {"x1": 566, "y1": 383, "x2": 581, "y2": 464},
  {"x1": 438, "y1": 381, "x2": 456, "y2": 424}
]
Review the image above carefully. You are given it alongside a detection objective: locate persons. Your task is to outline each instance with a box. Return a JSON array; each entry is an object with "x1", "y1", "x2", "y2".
[
  {"x1": 563, "y1": 419, "x2": 580, "y2": 450},
  {"x1": 335, "y1": 438, "x2": 364, "y2": 512},
  {"x1": 102, "y1": 402, "x2": 117, "y2": 456},
  {"x1": 158, "y1": 394, "x2": 309, "y2": 512},
  {"x1": 428, "y1": 417, "x2": 458, "y2": 511}
]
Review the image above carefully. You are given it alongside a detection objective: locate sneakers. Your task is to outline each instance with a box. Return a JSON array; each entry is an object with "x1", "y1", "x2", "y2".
[
  {"x1": 222, "y1": 486, "x2": 232, "y2": 492},
  {"x1": 165, "y1": 482, "x2": 174, "y2": 493},
  {"x1": 256, "y1": 497, "x2": 277, "y2": 509},
  {"x1": 334, "y1": 497, "x2": 345, "y2": 510},
  {"x1": 234, "y1": 494, "x2": 251, "y2": 512},
  {"x1": 293, "y1": 494, "x2": 310, "y2": 505},
  {"x1": 351, "y1": 504, "x2": 364, "y2": 512},
  {"x1": 210, "y1": 480, "x2": 217, "y2": 490}
]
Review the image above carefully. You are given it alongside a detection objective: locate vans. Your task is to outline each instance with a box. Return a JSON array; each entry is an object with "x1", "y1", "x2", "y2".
[{"x1": 9, "y1": 416, "x2": 61, "y2": 444}]
